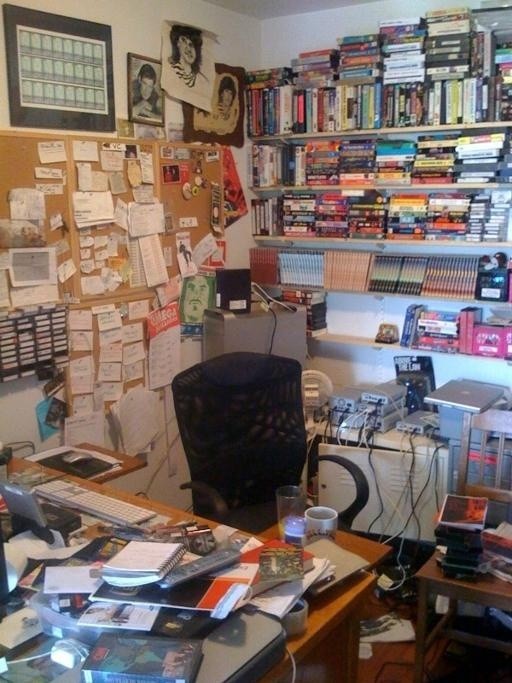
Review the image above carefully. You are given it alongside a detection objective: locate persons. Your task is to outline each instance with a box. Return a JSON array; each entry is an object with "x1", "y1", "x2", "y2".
[
  {"x1": 163, "y1": 166, "x2": 173, "y2": 181},
  {"x1": 194, "y1": 161, "x2": 202, "y2": 174},
  {"x1": 182, "y1": 275, "x2": 209, "y2": 324},
  {"x1": 165, "y1": 24, "x2": 210, "y2": 89},
  {"x1": 132, "y1": 63, "x2": 162, "y2": 121},
  {"x1": 213, "y1": 76, "x2": 237, "y2": 126},
  {"x1": 129, "y1": 151, "x2": 142, "y2": 186},
  {"x1": 171, "y1": 168, "x2": 179, "y2": 181},
  {"x1": 179, "y1": 241, "x2": 192, "y2": 267}
]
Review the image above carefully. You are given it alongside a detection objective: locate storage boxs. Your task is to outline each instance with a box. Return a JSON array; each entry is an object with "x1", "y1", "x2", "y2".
[{"x1": 439, "y1": 388, "x2": 511, "y2": 443}]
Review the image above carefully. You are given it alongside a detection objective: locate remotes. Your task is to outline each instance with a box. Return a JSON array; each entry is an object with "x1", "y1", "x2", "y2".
[{"x1": 154, "y1": 548, "x2": 242, "y2": 589}]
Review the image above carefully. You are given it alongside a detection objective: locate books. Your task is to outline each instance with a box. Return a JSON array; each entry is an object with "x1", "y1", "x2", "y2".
[
  {"x1": 283, "y1": 290, "x2": 328, "y2": 338},
  {"x1": 251, "y1": 190, "x2": 511, "y2": 244},
  {"x1": 249, "y1": 246, "x2": 512, "y2": 304},
  {"x1": 245, "y1": 133, "x2": 512, "y2": 189},
  {"x1": 431, "y1": 493, "x2": 511, "y2": 583},
  {"x1": 3, "y1": 525, "x2": 371, "y2": 683},
  {"x1": 400, "y1": 304, "x2": 512, "y2": 362},
  {"x1": 244, "y1": 1, "x2": 512, "y2": 138}
]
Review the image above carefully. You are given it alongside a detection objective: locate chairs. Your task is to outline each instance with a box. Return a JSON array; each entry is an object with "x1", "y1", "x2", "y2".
[
  {"x1": 415, "y1": 409, "x2": 511, "y2": 683},
  {"x1": 171, "y1": 352, "x2": 368, "y2": 537}
]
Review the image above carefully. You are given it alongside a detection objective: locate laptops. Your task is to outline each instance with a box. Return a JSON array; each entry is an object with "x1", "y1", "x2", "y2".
[{"x1": 422, "y1": 379, "x2": 506, "y2": 415}]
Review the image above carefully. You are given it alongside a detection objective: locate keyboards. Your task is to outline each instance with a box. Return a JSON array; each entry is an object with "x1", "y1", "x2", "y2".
[{"x1": 32, "y1": 480, "x2": 158, "y2": 525}]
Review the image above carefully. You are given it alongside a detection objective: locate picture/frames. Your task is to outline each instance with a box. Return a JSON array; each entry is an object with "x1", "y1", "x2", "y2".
[
  {"x1": 127, "y1": 52, "x2": 166, "y2": 126},
  {"x1": 181, "y1": 63, "x2": 245, "y2": 148},
  {"x1": 3, "y1": 2, "x2": 116, "y2": 133}
]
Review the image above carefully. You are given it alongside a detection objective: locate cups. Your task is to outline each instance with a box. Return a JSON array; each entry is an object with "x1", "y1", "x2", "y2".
[
  {"x1": 301, "y1": 506, "x2": 337, "y2": 543},
  {"x1": 274, "y1": 486, "x2": 307, "y2": 547}
]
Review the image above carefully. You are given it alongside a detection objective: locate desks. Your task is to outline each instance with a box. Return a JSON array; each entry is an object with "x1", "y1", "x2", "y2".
[{"x1": 0, "y1": 445, "x2": 375, "y2": 683}]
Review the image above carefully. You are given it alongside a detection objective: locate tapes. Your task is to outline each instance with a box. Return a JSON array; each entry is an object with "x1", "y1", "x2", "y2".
[{"x1": 281, "y1": 597, "x2": 310, "y2": 636}]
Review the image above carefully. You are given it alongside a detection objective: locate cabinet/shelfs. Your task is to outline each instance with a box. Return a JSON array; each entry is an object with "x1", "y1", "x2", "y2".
[
  {"x1": 247, "y1": 121, "x2": 512, "y2": 367},
  {"x1": 305, "y1": 419, "x2": 511, "y2": 547}
]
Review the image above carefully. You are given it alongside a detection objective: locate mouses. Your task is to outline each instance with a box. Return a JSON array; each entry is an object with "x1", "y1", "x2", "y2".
[{"x1": 61, "y1": 452, "x2": 93, "y2": 464}]
